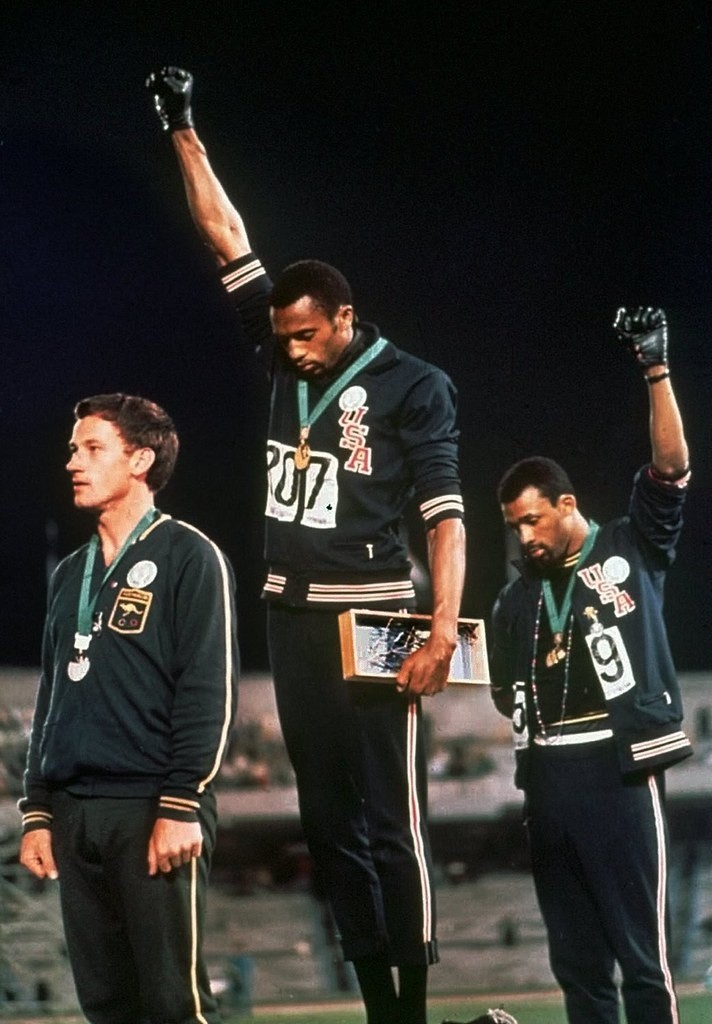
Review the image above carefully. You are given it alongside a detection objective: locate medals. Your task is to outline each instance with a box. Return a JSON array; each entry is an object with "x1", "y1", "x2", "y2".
[
  {"x1": 545, "y1": 646, "x2": 566, "y2": 668},
  {"x1": 294, "y1": 444, "x2": 311, "y2": 470},
  {"x1": 67, "y1": 655, "x2": 89, "y2": 681}
]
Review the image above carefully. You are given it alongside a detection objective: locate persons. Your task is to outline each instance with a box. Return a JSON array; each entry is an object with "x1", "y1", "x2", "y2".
[
  {"x1": 16, "y1": 395, "x2": 243, "y2": 1023},
  {"x1": 148, "y1": 65, "x2": 469, "y2": 1024},
  {"x1": 486, "y1": 305, "x2": 695, "y2": 1023}
]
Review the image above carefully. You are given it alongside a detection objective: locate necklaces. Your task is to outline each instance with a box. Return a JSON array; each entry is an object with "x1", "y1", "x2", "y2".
[{"x1": 531, "y1": 587, "x2": 574, "y2": 744}]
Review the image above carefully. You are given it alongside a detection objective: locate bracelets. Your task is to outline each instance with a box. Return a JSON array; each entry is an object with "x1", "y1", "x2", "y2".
[{"x1": 645, "y1": 370, "x2": 670, "y2": 384}]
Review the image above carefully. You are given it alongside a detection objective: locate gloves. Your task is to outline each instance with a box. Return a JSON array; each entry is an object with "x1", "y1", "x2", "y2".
[
  {"x1": 144, "y1": 64, "x2": 194, "y2": 132},
  {"x1": 612, "y1": 304, "x2": 669, "y2": 368}
]
